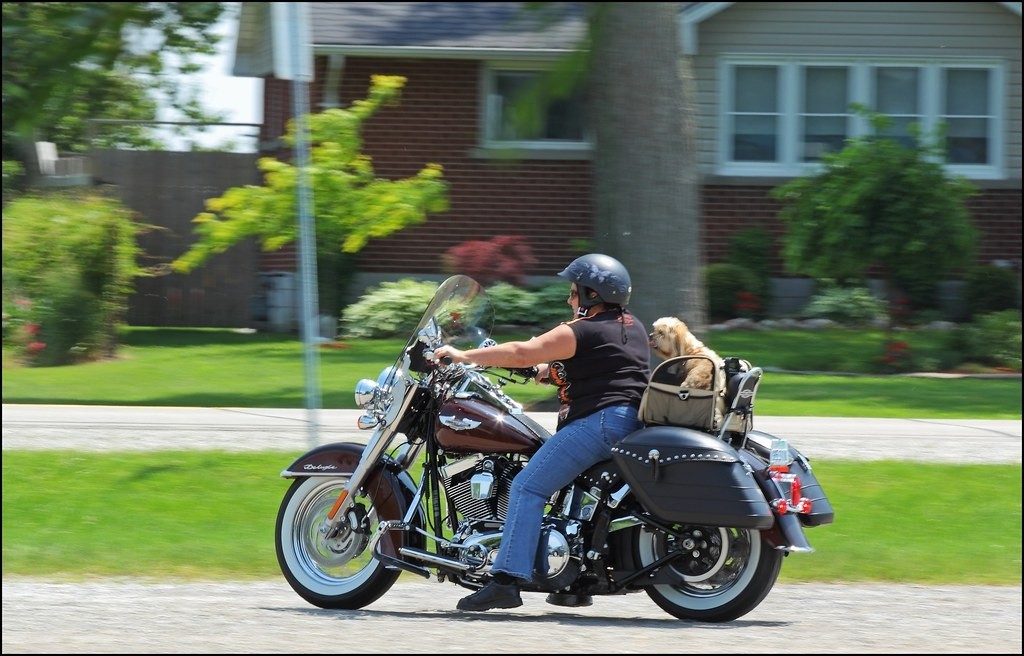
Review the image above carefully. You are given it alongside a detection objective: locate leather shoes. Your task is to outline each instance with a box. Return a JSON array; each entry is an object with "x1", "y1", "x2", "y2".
[
  {"x1": 546, "y1": 593, "x2": 592, "y2": 607},
  {"x1": 457, "y1": 580, "x2": 523, "y2": 611}
]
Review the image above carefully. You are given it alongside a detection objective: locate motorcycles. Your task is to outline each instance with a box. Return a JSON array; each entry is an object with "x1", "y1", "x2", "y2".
[{"x1": 274, "y1": 274, "x2": 837, "y2": 624}]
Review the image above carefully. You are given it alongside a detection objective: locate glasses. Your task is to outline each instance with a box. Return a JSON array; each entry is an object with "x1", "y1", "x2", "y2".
[{"x1": 569, "y1": 289, "x2": 578, "y2": 300}]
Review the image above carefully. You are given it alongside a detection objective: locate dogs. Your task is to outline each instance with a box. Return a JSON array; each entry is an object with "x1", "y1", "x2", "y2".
[{"x1": 648, "y1": 316, "x2": 726, "y2": 390}]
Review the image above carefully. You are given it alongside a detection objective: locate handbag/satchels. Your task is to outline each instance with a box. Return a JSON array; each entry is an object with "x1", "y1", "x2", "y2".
[{"x1": 637, "y1": 355, "x2": 755, "y2": 434}]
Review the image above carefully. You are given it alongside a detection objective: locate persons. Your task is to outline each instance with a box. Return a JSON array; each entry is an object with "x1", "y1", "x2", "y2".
[{"x1": 434, "y1": 253, "x2": 652, "y2": 612}]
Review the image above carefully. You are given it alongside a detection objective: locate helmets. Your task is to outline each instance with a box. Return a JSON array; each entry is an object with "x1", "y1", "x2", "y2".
[{"x1": 557, "y1": 253, "x2": 632, "y2": 307}]
